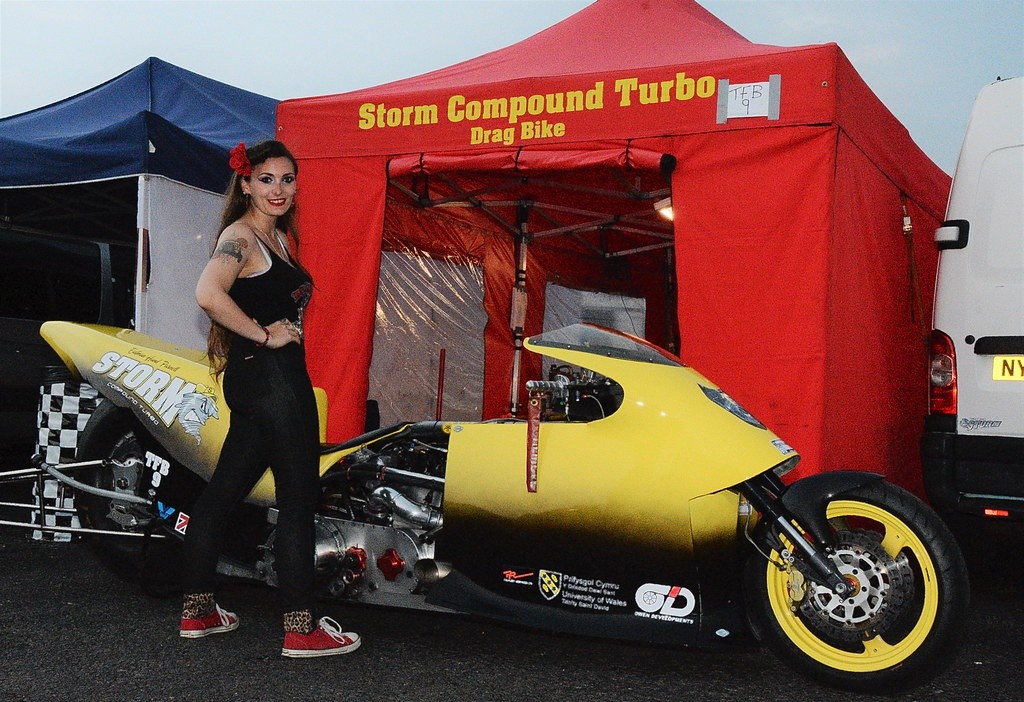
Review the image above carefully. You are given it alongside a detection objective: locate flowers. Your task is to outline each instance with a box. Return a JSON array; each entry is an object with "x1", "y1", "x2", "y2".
[{"x1": 228, "y1": 141, "x2": 252, "y2": 178}]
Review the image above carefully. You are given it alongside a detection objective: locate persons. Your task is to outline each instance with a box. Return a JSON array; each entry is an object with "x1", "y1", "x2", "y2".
[{"x1": 178, "y1": 137, "x2": 363, "y2": 657}]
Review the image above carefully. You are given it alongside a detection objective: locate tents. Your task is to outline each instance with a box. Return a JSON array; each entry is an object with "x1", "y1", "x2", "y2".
[{"x1": 0, "y1": 0, "x2": 954, "y2": 550}]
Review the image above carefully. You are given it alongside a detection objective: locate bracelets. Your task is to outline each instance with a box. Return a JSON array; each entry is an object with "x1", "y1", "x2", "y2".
[{"x1": 254, "y1": 327, "x2": 270, "y2": 348}]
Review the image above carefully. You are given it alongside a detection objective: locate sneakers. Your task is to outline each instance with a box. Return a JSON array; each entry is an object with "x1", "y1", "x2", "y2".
[
  {"x1": 281, "y1": 609, "x2": 361, "y2": 658},
  {"x1": 180, "y1": 593, "x2": 240, "y2": 638}
]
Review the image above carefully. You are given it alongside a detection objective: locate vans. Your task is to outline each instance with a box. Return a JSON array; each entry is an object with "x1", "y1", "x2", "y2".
[{"x1": 917, "y1": 71, "x2": 1023, "y2": 539}]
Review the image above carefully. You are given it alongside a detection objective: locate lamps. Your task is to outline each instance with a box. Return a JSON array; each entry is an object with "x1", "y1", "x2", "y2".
[{"x1": 653, "y1": 197, "x2": 674, "y2": 222}]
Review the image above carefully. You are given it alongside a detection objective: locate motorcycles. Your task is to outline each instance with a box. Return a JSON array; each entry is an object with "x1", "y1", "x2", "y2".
[{"x1": 1, "y1": 318, "x2": 979, "y2": 699}]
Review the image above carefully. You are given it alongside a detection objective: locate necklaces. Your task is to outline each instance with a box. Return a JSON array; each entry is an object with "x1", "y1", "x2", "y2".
[{"x1": 246, "y1": 208, "x2": 285, "y2": 259}]
